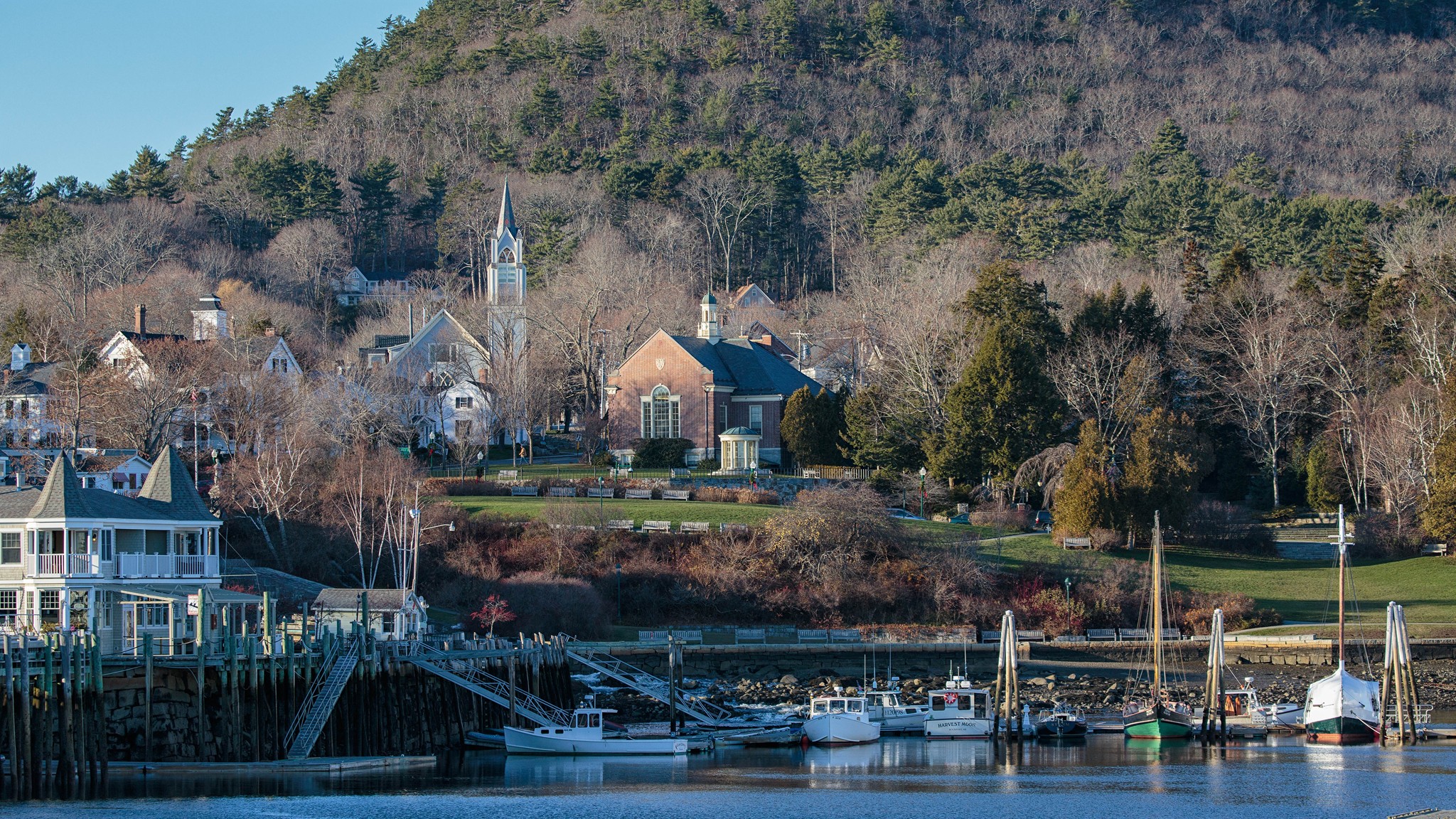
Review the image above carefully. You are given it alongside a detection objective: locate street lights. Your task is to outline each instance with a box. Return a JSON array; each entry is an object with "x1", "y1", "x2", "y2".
[
  {"x1": 920, "y1": 467, "x2": 926, "y2": 517},
  {"x1": 598, "y1": 476, "x2": 604, "y2": 529},
  {"x1": 600, "y1": 358, "x2": 620, "y2": 455},
  {"x1": 614, "y1": 563, "x2": 622, "y2": 622},
  {"x1": 429, "y1": 432, "x2": 435, "y2": 471},
  {"x1": 750, "y1": 460, "x2": 756, "y2": 491},
  {"x1": 477, "y1": 452, "x2": 483, "y2": 483},
  {"x1": 1064, "y1": 578, "x2": 1071, "y2": 632},
  {"x1": 409, "y1": 509, "x2": 455, "y2": 595},
  {"x1": 780, "y1": 353, "x2": 802, "y2": 372},
  {"x1": 369, "y1": 426, "x2": 374, "y2": 445}
]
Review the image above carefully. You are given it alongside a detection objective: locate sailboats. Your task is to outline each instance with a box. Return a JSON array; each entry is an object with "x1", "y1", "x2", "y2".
[
  {"x1": 1122, "y1": 510, "x2": 1194, "y2": 741},
  {"x1": 1302, "y1": 505, "x2": 1380, "y2": 746}
]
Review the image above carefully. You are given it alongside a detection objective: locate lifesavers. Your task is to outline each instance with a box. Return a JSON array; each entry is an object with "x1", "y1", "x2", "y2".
[
  {"x1": 944, "y1": 692, "x2": 956, "y2": 704},
  {"x1": 821, "y1": 696, "x2": 831, "y2": 698},
  {"x1": 1184, "y1": 707, "x2": 1190, "y2": 715}
]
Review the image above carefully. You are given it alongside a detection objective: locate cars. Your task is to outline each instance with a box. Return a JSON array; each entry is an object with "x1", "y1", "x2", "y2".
[
  {"x1": 887, "y1": 508, "x2": 927, "y2": 520},
  {"x1": 948, "y1": 513, "x2": 973, "y2": 525}
]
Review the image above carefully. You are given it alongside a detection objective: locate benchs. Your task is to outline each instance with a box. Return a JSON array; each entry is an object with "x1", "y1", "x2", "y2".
[
  {"x1": 680, "y1": 521, "x2": 709, "y2": 533},
  {"x1": 607, "y1": 520, "x2": 634, "y2": 531},
  {"x1": 1087, "y1": 629, "x2": 1117, "y2": 641},
  {"x1": 1421, "y1": 544, "x2": 1448, "y2": 556},
  {"x1": 662, "y1": 489, "x2": 690, "y2": 501},
  {"x1": 499, "y1": 470, "x2": 518, "y2": 480},
  {"x1": 797, "y1": 629, "x2": 828, "y2": 644},
  {"x1": 641, "y1": 520, "x2": 671, "y2": 533},
  {"x1": 1152, "y1": 628, "x2": 1181, "y2": 640},
  {"x1": 719, "y1": 522, "x2": 748, "y2": 534},
  {"x1": 1062, "y1": 537, "x2": 1092, "y2": 550},
  {"x1": 610, "y1": 469, "x2": 628, "y2": 478},
  {"x1": 803, "y1": 470, "x2": 819, "y2": 478},
  {"x1": 624, "y1": 488, "x2": 652, "y2": 500},
  {"x1": 636, "y1": 631, "x2": 669, "y2": 642},
  {"x1": 1118, "y1": 628, "x2": 1149, "y2": 640},
  {"x1": 735, "y1": 629, "x2": 766, "y2": 644},
  {"x1": 671, "y1": 468, "x2": 691, "y2": 478},
  {"x1": 512, "y1": 486, "x2": 538, "y2": 496},
  {"x1": 1016, "y1": 630, "x2": 1046, "y2": 642},
  {"x1": 754, "y1": 469, "x2": 773, "y2": 478},
  {"x1": 844, "y1": 470, "x2": 858, "y2": 478},
  {"x1": 830, "y1": 629, "x2": 861, "y2": 644},
  {"x1": 981, "y1": 631, "x2": 1000, "y2": 643},
  {"x1": 588, "y1": 488, "x2": 615, "y2": 499},
  {"x1": 549, "y1": 486, "x2": 576, "y2": 497},
  {"x1": 671, "y1": 630, "x2": 703, "y2": 645}
]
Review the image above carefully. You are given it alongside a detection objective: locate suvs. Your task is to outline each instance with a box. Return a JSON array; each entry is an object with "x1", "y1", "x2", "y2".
[{"x1": 1035, "y1": 510, "x2": 1058, "y2": 533}]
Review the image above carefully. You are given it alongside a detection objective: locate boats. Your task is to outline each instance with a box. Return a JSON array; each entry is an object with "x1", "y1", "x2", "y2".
[
  {"x1": 1192, "y1": 676, "x2": 1305, "y2": 734},
  {"x1": 803, "y1": 654, "x2": 881, "y2": 747},
  {"x1": 1034, "y1": 698, "x2": 1088, "y2": 739},
  {"x1": 922, "y1": 635, "x2": 1005, "y2": 740},
  {"x1": 464, "y1": 728, "x2": 506, "y2": 749},
  {"x1": 785, "y1": 621, "x2": 929, "y2": 735},
  {"x1": 503, "y1": 689, "x2": 688, "y2": 756}
]
